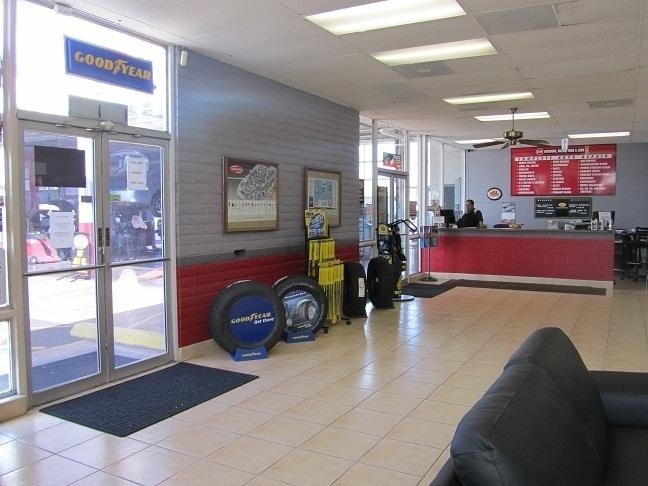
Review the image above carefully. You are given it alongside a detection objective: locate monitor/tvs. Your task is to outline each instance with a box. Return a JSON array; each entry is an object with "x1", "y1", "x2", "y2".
[{"x1": 440, "y1": 209, "x2": 455, "y2": 227}]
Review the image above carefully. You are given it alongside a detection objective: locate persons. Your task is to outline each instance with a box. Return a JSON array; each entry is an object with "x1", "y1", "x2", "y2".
[{"x1": 456, "y1": 199, "x2": 483, "y2": 228}]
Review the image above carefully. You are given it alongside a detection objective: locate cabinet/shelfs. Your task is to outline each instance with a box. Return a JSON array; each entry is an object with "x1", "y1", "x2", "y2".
[{"x1": 620, "y1": 227, "x2": 648, "y2": 283}]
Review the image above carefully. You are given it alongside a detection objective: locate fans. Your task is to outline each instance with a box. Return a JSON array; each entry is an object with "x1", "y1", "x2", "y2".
[{"x1": 470, "y1": 103, "x2": 554, "y2": 153}]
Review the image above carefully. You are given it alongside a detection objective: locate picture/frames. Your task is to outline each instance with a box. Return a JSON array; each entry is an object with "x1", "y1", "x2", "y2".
[
  {"x1": 302, "y1": 164, "x2": 343, "y2": 230},
  {"x1": 220, "y1": 152, "x2": 281, "y2": 235}
]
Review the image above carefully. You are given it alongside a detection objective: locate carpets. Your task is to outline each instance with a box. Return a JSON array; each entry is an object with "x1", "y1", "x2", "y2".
[
  {"x1": 401, "y1": 280, "x2": 451, "y2": 299},
  {"x1": 452, "y1": 275, "x2": 607, "y2": 298},
  {"x1": 38, "y1": 357, "x2": 261, "y2": 439}
]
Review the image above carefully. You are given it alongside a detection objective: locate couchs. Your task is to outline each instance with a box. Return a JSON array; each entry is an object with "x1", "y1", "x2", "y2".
[{"x1": 427, "y1": 323, "x2": 646, "y2": 485}]
